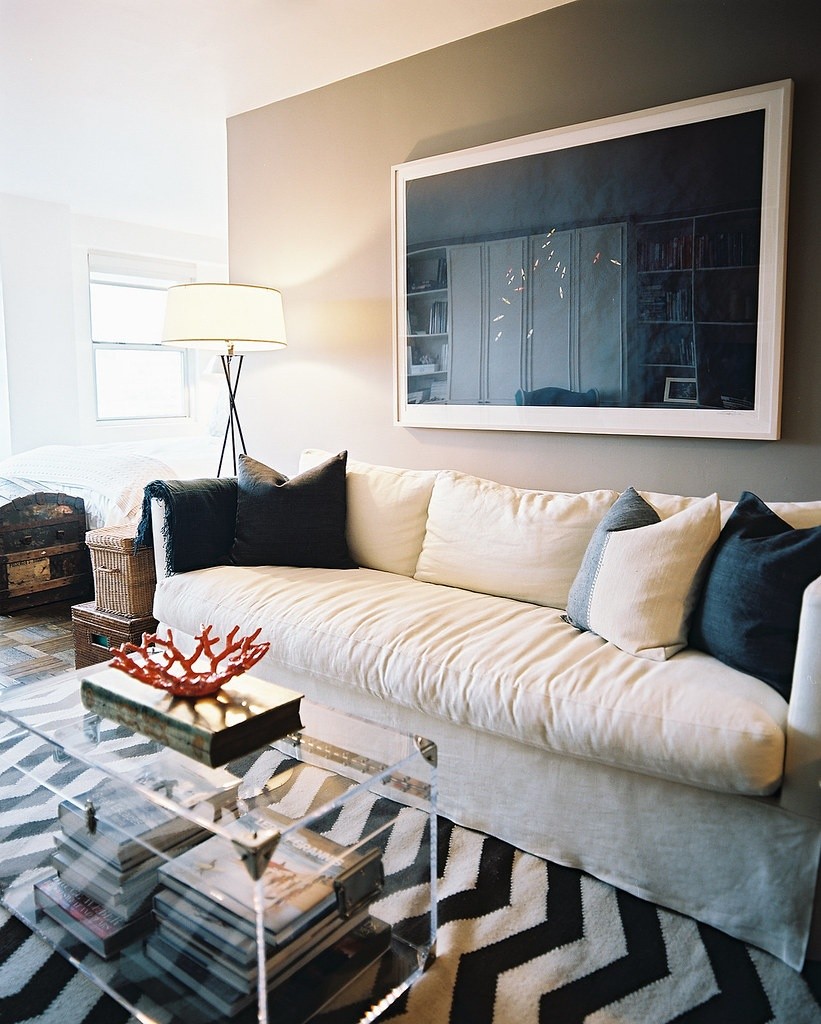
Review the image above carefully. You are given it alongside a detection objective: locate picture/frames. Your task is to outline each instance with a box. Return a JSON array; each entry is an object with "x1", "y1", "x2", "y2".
[{"x1": 664, "y1": 377, "x2": 697, "y2": 404}]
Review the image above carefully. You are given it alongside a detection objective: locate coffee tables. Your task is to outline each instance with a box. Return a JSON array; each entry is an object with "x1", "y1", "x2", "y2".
[{"x1": 0, "y1": 646, "x2": 438, "y2": 1024}]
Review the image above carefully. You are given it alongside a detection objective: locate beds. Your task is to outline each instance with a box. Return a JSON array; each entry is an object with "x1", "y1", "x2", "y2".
[{"x1": 0, "y1": 435, "x2": 210, "y2": 616}]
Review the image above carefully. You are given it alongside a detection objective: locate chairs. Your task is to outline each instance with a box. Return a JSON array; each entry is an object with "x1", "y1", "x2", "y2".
[{"x1": 515, "y1": 387, "x2": 600, "y2": 407}]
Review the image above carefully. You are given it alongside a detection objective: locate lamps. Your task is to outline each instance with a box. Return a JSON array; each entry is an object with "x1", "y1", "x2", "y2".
[{"x1": 161, "y1": 283, "x2": 288, "y2": 478}]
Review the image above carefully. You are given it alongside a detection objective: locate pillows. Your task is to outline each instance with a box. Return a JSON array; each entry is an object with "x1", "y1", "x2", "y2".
[
  {"x1": 685, "y1": 492, "x2": 821, "y2": 706},
  {"x1": 230, "y1": 450, "x2": 360, "y2": 570},
  {"x1": 559, "y1": 486, "x2": 722, "y2": 663}
]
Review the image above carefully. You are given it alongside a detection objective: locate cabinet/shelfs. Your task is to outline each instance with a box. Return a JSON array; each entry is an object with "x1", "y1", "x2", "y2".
[{"x1": 406, "y1": 201, "x2": 761, "y2": 411}]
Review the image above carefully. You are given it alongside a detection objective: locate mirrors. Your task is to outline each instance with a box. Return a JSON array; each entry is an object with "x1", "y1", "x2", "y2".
[{"x1": 390, "y1": 79, "x2": 794, "y2": 440}]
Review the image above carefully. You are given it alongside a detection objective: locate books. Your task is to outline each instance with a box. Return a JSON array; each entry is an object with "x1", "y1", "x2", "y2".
[
  {"x1": 406, "y1": 254, "x2": 452, "y2": 402},
  {"x1": 34, "y1": 755, "x2": 392, "y2": 1024},
  {"x1": 634, "y1": 226, "x2": 759, "y2": 409}
]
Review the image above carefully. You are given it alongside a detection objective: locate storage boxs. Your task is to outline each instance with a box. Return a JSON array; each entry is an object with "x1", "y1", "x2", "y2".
[
  {"x1": 411, "y1": 364, "x2": 439, "y2": 374},
  {"x1": 85, "y1": 522, "x2": 156, "y2": 619},
  {"x1": 70, "y1": 602, "x2": 160, "y2": 670}
]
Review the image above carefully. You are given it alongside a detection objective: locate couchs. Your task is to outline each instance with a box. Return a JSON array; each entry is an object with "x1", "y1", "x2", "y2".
[{"x1": 131, "y1": 449, "x2": 821, "y2": 973}]
